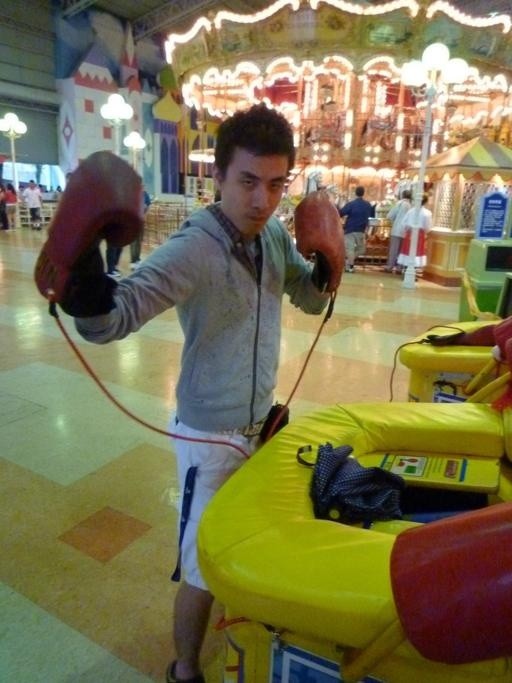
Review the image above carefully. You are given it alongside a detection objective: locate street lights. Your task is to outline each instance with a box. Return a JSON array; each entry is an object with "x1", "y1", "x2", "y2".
[
  {"x1": 0, "y1": 113, "x2": 27, "y2": 229},
  {"x1": 400, "y1": 41, "x2": 470, "y2": 291},
  {"x1": 100, "y1": 93, "x2": 147, "y2": 171}
]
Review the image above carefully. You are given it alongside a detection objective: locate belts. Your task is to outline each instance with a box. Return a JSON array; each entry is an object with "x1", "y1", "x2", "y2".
[{"x1": 215, "y1": 416, "x2": 266, "y2": 436}]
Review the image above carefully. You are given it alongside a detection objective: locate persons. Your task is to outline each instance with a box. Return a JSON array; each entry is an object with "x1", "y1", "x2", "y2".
[
  {"x1": 30, "y1": 102, "x2": 350, "y2": 681},
  {"x1": 104, "y1": 240, "x2": 123, "y2": 278},
  {"x1": 333, "y1": 185, "x2": 376, "y2": 274},
  {"x1": 396, "y1": 193, "x2": 433, "y2": 278},
  {"x1": 1, "y1": 179, "x2": 62, "y2": 232},
  {"x1": 378, "y1": 189, "x2": 414, "y2": 274},
  {"x1": 127, "y1": 174, "x2": 152, "y2": 271}
]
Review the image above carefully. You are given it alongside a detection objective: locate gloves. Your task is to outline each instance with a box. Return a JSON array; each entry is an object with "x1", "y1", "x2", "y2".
[
  {"x1": 292, "y1": 190, "x2": 349, "y2": 294},
  {"x1": 32, "y1": 146, "x2": 148, "y2": 318}
]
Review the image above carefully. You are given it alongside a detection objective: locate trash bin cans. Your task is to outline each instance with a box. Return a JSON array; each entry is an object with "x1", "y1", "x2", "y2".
[{"x1": 459, "y1": 238, "x2": 512, "y2": 322}]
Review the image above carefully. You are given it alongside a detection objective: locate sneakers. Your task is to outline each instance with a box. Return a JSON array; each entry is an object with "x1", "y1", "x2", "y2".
[{"x1": 165, "y1": 657, "x2": 207, "y2": 683}]
